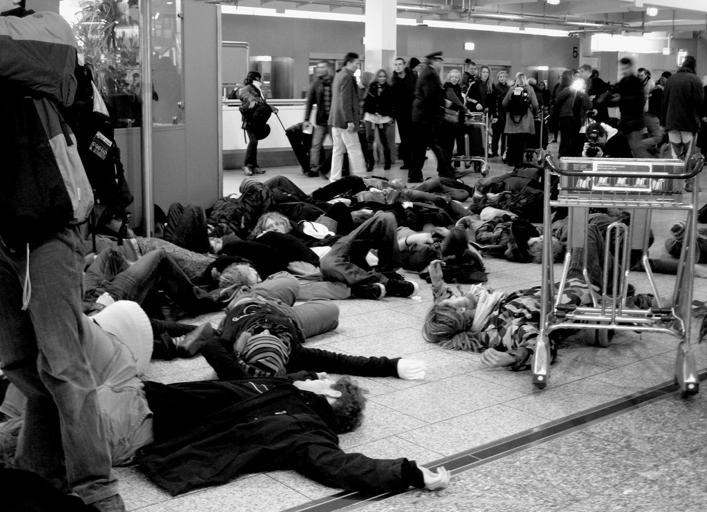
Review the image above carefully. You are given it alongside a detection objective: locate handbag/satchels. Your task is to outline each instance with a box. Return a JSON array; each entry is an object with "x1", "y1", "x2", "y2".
[
  {"x1": 240, "y1": 97, "x2": 272, "y2": 127},
  {"x1": 246, "y1": 124, "x2": 273, "y2": 140}
]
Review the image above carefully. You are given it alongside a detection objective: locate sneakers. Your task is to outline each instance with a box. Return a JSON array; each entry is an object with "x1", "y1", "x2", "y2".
[
  {"x1": 254, "y1": 167, "x2": 267, "y2": 175},
  {"x1": 368, "y1": 160, "x2": 462, "y2": 184},
  {"x1": 372, "y1": 266, "x2": 416, "y2": 297},
  {"x1": 241, "y1": 166, "x2": 255, "y2": 175}
]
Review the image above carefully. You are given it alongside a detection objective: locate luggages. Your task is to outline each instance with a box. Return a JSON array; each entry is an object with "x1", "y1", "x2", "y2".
[{"x1": 285, "y1": 122, "x2": 331, "y2": 175}]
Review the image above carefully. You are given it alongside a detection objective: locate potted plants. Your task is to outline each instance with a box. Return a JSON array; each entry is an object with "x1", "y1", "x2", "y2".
[{"x1": 69, "y1": 1, "x2": 142, "y2": 128}]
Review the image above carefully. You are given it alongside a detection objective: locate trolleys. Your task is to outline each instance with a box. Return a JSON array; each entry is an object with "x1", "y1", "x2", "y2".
[
  {"x1": 449, "y1": 106, "x2": 495, "y2": 178},
  {"x1": 513, "y1": 138, "x2": 702, "y2": 403},
  {"x1": 508, "y1": 106, "x2": 549, "y2": 164}
]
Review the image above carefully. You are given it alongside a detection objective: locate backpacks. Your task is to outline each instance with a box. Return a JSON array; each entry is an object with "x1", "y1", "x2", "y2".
[{"x1": 510, "y1": 84, "x2": 530, "y2": 119}]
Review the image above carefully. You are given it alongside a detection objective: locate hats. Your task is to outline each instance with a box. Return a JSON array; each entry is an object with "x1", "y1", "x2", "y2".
[
  {"x1": 670, "y1": 219, "x2": 701, "y2": 245},
  {"x1": 240, "y1": 335, "x2": 289, "y2": 381},
  {"x1": 425, "y1": 50, "x2": 444, "y2": 61},
  {"x1": 683, "y1": 55, "x2": 699, "y2": 70},
  {"x1": 584, "y1": 122, "x2": 604, "y2": 142}
]
